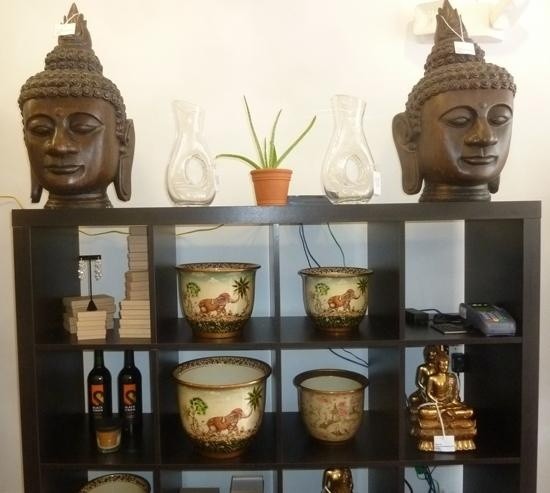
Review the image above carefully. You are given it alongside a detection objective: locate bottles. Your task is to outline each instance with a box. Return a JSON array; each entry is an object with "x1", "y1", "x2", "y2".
[{"x1": 86, "y1": 349, "x2": 144, "y2": 436}]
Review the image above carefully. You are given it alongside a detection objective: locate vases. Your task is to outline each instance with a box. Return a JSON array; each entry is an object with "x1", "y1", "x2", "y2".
[
  {"x1": 297, "y1": 265, "x2": 374, "y2": 339},
  {"x1": 80, "y1": 473, "x2": 151, "y2": 492},
  {"x1": 166, "y1": 99, "x2": 218, "y2": 207},
  {"x1": 170, "y1": 261, "x2": 261, "y2": 339},
  {"x1": 292, "y1": 368, "x2": 370, "y2": 446},
  {"x1": 171, "y1": 355, "x2": 272, "y2": 461},
  {"x1": 320, "y1": 93, "x2": 376, "y2": 204}
]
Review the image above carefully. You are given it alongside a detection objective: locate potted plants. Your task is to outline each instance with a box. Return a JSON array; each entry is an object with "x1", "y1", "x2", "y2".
[{"x1": 213, "y1": 94, "x2": 316, "y2": 206}]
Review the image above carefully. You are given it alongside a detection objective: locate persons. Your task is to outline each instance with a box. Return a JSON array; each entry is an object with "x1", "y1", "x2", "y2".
[
  {"x1": 322, "y1": 468, "x2": 353, "y2": 493},
  {"x1": 393, "y1": 60, "x2": 517, "y2": 203},
  {"x1": 18, "y1": 69, "x2": 135, "y2": 209},
  {"x1": 408, "y1": 345, "x2": 475, "y2": 423}
]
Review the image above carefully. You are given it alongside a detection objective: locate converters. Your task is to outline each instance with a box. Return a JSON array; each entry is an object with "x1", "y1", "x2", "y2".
[{"x1": 405, "y1": 308, "x2": 428, "y2": 327}]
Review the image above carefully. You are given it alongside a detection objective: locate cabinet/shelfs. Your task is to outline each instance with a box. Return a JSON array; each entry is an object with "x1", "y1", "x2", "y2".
[{"x1": 11, "y1": 202, "x2": 542, "y2": 492}]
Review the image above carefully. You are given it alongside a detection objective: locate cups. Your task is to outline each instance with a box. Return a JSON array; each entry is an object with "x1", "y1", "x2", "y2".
[{"x1": 94, "y1": 416, "x2": 122, "y2": 453}]
